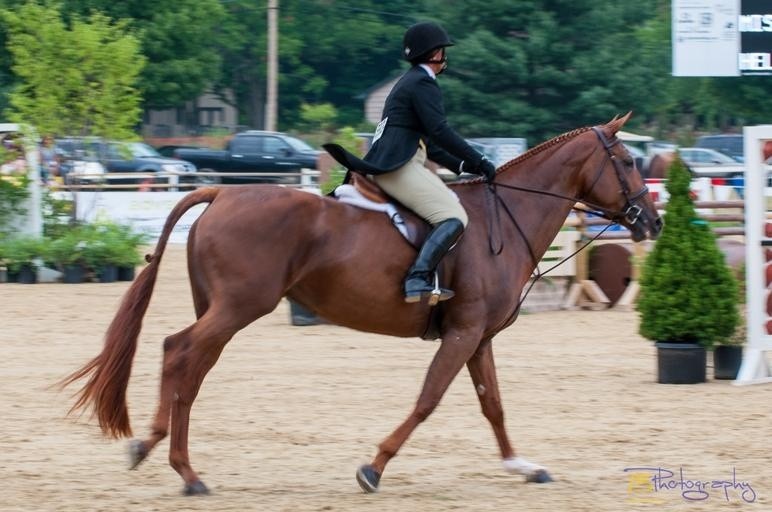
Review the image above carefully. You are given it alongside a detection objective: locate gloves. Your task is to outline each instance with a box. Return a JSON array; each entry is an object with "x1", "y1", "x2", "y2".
[
  {"x1": 461, "y1": 161, "x2": 483, "y2": 176},
  {"x1": 481, "y1": 159, "x2": 496, "y2": 184}
]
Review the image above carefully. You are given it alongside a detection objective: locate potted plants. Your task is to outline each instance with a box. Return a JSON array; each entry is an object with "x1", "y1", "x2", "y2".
[
  {"x1": 3, "y1": 227, "x2": 145, "y2": 282},
  {"x1": 640, "y1": 147, "x2": 744, "y2": 383}
]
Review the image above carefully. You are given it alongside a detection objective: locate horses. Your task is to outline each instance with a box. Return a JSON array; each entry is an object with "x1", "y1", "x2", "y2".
[{"x1": 54, "y1": 109, "x2": 664, "y2": 495}]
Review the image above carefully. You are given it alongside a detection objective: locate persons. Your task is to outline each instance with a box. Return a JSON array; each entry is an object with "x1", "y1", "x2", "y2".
[
  {"x1": 44, "y1": 163, "x2": 64, "y2": 190},
  {"x1": 291, "y1": 21, "x2": 495, "y2": 326},
  {"x1": 137, "y1": 168, "x2": 163, "y2": 193},
  {"x1": 39, "y1": 133, "x2": 68, "y2": 175}
]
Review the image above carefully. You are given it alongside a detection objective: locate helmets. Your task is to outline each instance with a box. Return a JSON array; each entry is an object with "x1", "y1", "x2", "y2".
[{"x1": 403, "y1": 20, "x2": 455, "y2": 61}]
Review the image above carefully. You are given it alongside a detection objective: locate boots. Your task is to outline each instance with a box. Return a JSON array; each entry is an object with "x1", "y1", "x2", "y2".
[{"x1": 404, "y1": 218, "x2": 464, "y2": 303}]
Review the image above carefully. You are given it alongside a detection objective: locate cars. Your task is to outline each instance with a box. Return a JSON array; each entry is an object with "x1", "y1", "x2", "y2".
[
  {"x1": 355, "y1": 118, "x2": 772, "y2": 193},
  {"x1": 0, "y1": 122, "x2": 329, "y2": 192}
]
